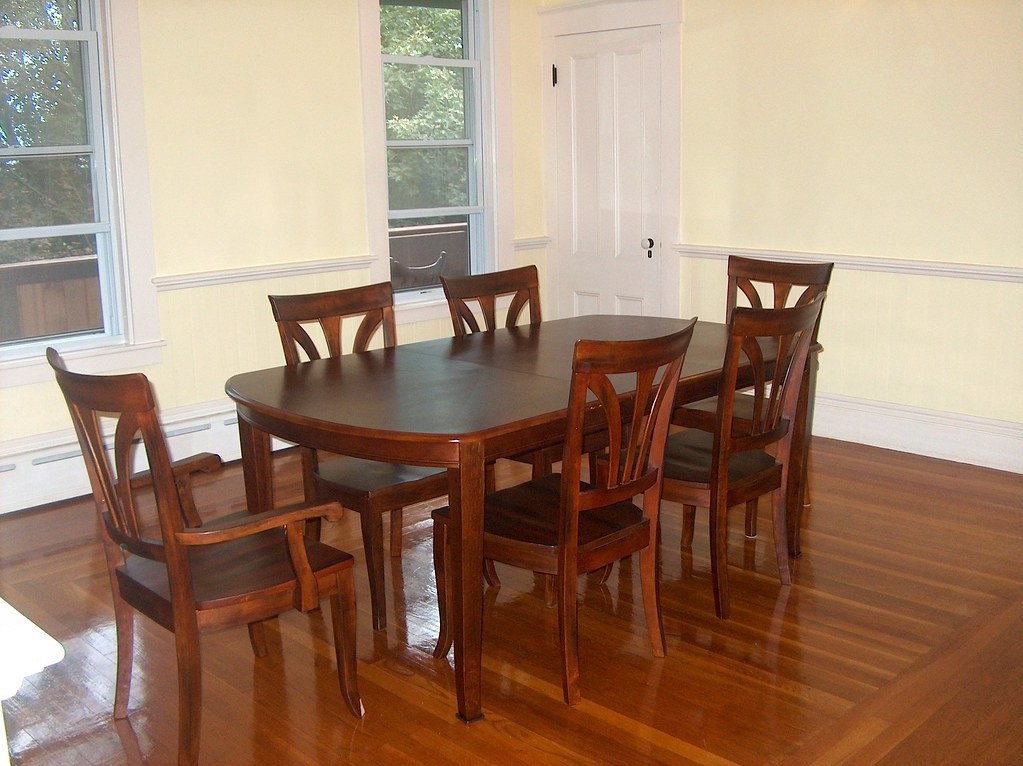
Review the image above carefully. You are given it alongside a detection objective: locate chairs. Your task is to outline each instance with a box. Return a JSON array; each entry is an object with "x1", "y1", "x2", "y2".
[
  {"x1": 439, "y1": 264, "x2": 609, "y2": 499},
  {"x1": 595, "y1": 289, "x2": 829, "y2": 623},
  {"x1": 670, "y1": 254, "x2": 834, "y2": 550},
  {"x1": 267, "y1": 281, "x2": 501, "y2": 633},
  {"x1": 45, "y1": 345, "x2": 366, "y2": 766},
  {"x1": 429, "y1": 317, "x2": 699, "y2": 705}
]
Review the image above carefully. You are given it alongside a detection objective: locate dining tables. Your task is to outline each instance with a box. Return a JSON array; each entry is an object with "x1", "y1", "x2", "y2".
[{"x1": 221, "y1": 316, "x2": 825, "y2": 726}]
